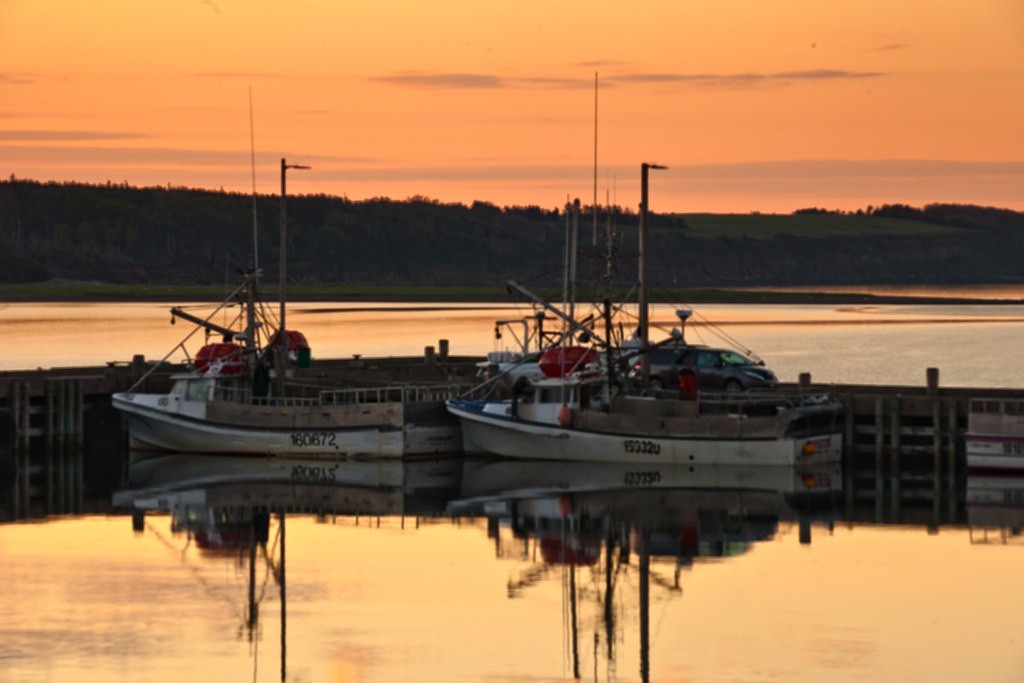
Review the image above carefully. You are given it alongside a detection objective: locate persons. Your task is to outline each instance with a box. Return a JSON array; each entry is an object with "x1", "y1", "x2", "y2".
[
  {"x1": 680, "y1": 359, "x2": 700, "y2": 402},
  {"x1": 512, "y1": 376, "x2": 532, "y2": 419},
  {"x1": 253, "y1": 360, "x2": 271, "y2": 406}
]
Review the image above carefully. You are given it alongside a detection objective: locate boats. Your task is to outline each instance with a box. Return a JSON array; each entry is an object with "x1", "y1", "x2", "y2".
[
  {"x1": 432, "y1": 69, "x2": 851, "y2": 498},
  {"x1": 110, "y1": 84, "x2": 459, "y2": 458},
  {"x1": 446, "y1": 457, "x2": 852, "y2": 683},
  {"x1": 110, "y1": 453, "x2": 455, "y2": 682}
]
